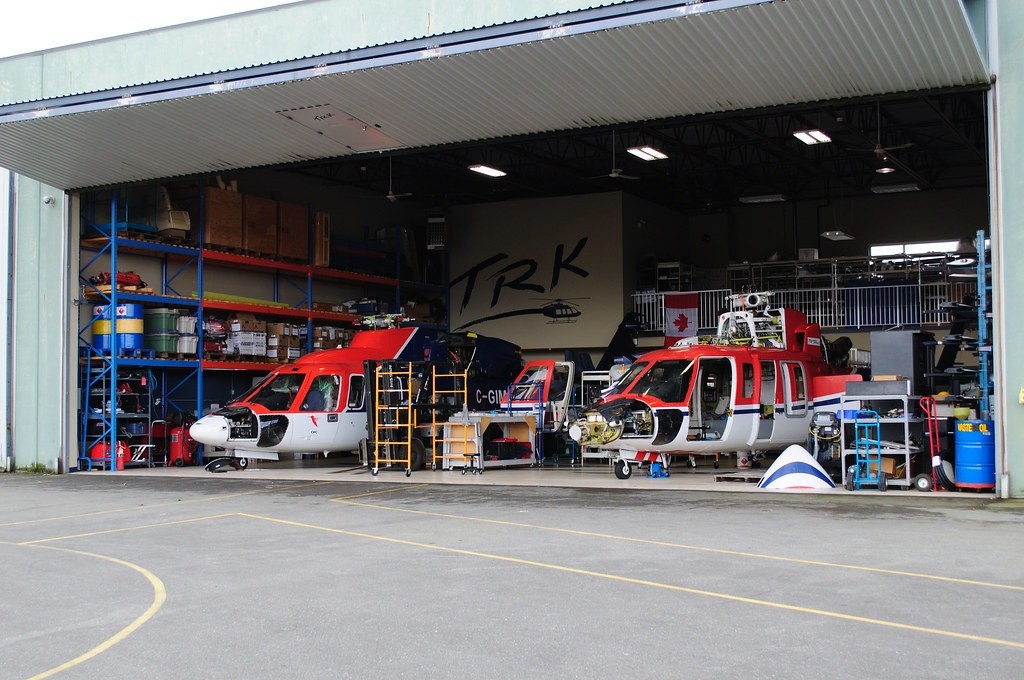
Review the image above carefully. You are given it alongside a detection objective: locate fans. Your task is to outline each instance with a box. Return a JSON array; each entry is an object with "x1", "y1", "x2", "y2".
[
  {"x1": 847, "y1": 102, "x2": 913, "y2": 158},
  {"x1": 588, "y1": 130, "x2": 640, "y2": 180},
  {"x1": 385, "y1": 155, "x2": 413, "y2": 202}
]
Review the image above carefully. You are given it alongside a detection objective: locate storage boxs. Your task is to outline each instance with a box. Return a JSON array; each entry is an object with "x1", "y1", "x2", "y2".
[
  {"x1": 846, "y1": 381, "x2": 907, "y2": 394},
  {"x1": 870, "y1": 455, "x2": 896, "y2": 475},
  {"x1": 186, "y1": 186, "x2": 308, "y2": 259},
  {"x1": 406, "y1": 304, "x2": 431, "y2": 318},
  {"x1": 871, "y1": 374, "x2": 902, "y2": 381},
  {"x1": 490, "y1": 442, "x2": 516, "y2": 460},
  {"x1": 145, "y1": 309, "x2": 363, "y2": 363}
]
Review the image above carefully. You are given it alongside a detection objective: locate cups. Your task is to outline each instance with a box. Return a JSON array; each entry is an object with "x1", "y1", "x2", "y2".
[{"x1": 117, "y1": 453, "x2": 124, "y2": 470}]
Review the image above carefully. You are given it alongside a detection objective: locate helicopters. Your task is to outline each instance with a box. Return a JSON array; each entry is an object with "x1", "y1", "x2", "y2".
[
  {"x1": 567, "y1": 283, "x2": 977, "y2": 480},
  {"x1": 187, "y1": 309, "x2": 642, "y2": 467}
]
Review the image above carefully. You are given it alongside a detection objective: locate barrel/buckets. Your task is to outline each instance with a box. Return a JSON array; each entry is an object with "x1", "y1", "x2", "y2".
[
  {"x1": 954, "y1": 420, "x2": 995, "y2": 488},
  {"x1": 737, "y1": 450, "x2": 752, "y2": 469}
]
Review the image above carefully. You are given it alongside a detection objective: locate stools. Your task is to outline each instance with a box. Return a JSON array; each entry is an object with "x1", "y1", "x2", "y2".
[{"x1": 462, "y1": 453, "x2": 482, "y2": 475}]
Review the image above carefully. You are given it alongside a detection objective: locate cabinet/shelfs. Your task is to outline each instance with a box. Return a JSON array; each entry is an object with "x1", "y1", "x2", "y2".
[
  {"x1": 657, "y1": 261, "x2": 692, "y2": 325},
  {"x1": 840, "y1": 395, "x2": 924, "y2": 490},
  {"x1": 726, "y1": 250, "x2": 992, "y2": 316},
  {"x1": 78, "y1": 175, "x2": 450, "y2": 479},
  {"x1": 922, "y1": 263, "x2": 979, "y2": 438}
]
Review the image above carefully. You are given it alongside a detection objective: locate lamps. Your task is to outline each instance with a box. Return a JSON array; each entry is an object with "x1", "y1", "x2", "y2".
[
  {"x1": 821, "y1": 230, "x2": 855, "y2": 240},
  {"x1": 627, "y1": 132, "x2": 669, "y2": 161},
  {"x1": 793, "y1": 108, "x2": 832, "y2": 146}
]
